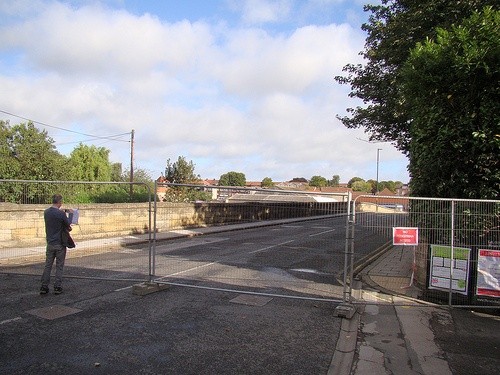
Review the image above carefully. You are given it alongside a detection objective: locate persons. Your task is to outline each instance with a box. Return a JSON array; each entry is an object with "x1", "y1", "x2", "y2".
[{"x1": 40, "y1": 194, "x2": 73, "y2": 293}]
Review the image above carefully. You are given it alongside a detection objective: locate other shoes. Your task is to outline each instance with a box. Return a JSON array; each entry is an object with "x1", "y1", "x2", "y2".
[
  {"x1": 53, "y1": 287, "x2": 65, "y2": 294},
  {"x1": 40, "y1": 287, "x2": 50, "y2": 294}
]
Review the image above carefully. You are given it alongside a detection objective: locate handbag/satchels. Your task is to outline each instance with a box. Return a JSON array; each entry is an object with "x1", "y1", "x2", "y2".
[{"x1": 61, "y1": 226, "x2": 75, "y2": 249}]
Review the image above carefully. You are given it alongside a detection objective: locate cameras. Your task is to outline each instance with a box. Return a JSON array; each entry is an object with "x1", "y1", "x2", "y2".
[{"x1": 65, "y1": 209, "x2": 69, "y2": 212}]
